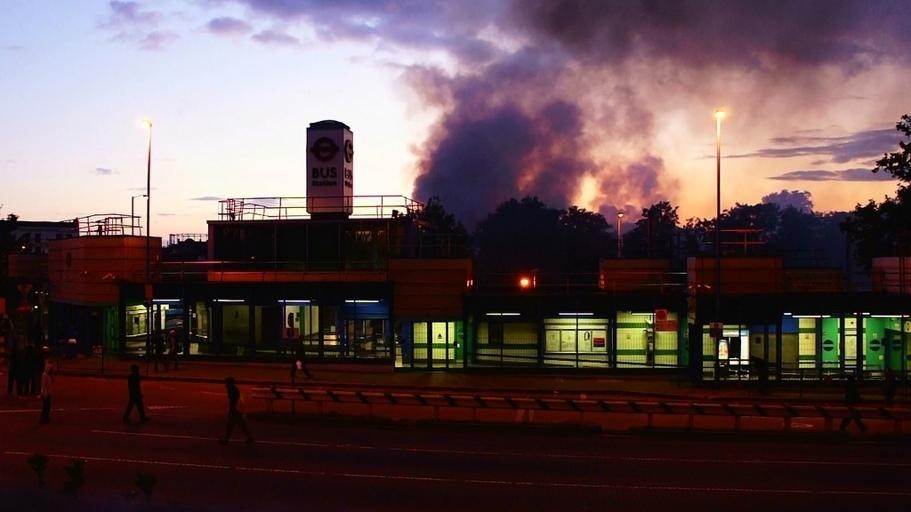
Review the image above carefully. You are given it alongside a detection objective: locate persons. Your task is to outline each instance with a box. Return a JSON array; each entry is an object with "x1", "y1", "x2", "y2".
[
  {"x1": 292, "y1": 333, "x2": 320, "y2": 379},
  {"x1": 38, "y1": 361, "x2": 54, "y2": 425},
  {"x1": 152, "y1": 324, "x2": 168, "y2": 370},
  {"x1": 869, "y1": 366, "x2": 896, "y2": 405},
  {"x1": 123, "y1": 361, "x2": 152, "y2": 424},
  {"x1": 8, "y1": 330, "x2": 48, "y2": 399},
  {"x1": 840, "y1": 372, "x2": 870, "y2": 434},
  {"x1": 167, "y1": 327, "x2": 179, "y2": 369},
  {"x1": 219, "y1": 375, "x2": 260, "y2": 445}
]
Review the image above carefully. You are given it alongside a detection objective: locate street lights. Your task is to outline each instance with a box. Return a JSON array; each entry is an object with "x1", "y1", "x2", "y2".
[
  {"x1": 142, "y1": 116, "x2": 156, "y2": 356},
  {"x1": 616, "y1": 211, "x2": 626, "y2": 259},
  {"x1": 712, "y1": 109, "x2": 727, "y2": 383}
]
